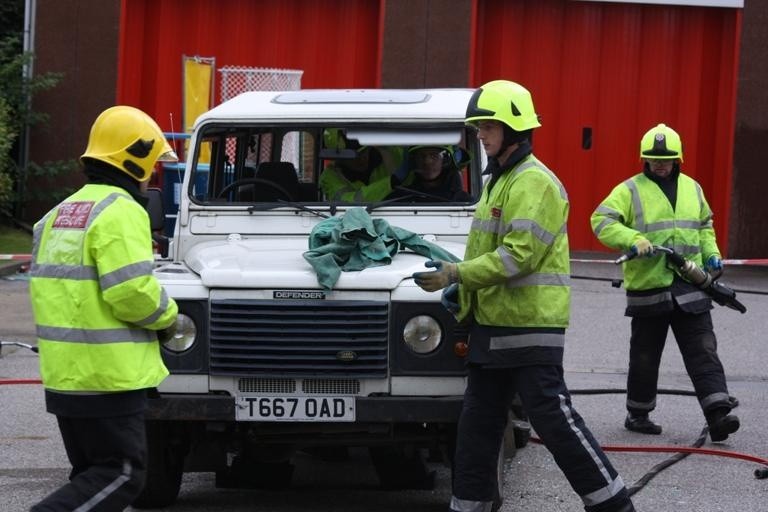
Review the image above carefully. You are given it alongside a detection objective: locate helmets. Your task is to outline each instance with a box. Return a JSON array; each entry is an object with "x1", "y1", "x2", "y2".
[
  {"x1": 407, "y1": 145, "x2": 453, "y2": 155},
  {"x1": 463, "y1": 78, "x2": 541, "y2": 132},
  {"x1": 323, "y1": 128, "x2": 366, "y2": 152},
  {"x1": 78, "y1": 105, "x2": 179, "y2": 183},
  {"x1": 639, "y1": 123, "x2": 683, "y2": 164}
]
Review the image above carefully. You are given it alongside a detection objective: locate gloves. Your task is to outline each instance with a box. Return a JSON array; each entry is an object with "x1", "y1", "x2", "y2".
[
  {"x1": 630, "y1": 239, "x2": 653, "y2": 258},
  {"x1": 703, "y1": 256, "x2": 722, "y2": 272},
  {"x1": 440, "y1": 283, "x2": 460, "y2": 314},
  {"x1": 412, "y1": 259, "x2": 457, "y2": 292}
]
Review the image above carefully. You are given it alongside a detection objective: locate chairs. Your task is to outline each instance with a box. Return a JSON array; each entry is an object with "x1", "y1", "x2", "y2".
[{"x1": 238, "y1": 162, "x2": 305, "y2": 201}]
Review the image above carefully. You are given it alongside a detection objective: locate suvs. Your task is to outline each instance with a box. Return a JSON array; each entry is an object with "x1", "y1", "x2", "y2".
[{"x1": 130, "y1": 86, "x2": 529, "y2": 510}]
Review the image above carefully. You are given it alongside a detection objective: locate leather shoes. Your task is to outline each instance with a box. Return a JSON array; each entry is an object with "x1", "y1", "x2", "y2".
[
  {"x1": 624, "y1": 410, "x2": 661, "y2": 434},
  {"x1": 705, "y1": 411, "x2": 738, "y2": 441}
]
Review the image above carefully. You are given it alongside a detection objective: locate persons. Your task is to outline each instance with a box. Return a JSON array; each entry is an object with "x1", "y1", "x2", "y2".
[
  {"x1": 316, "y1": 126, "x2": 476, "y2": 206},
  {"x1": 30, "y1": 104, "x2": 182, "y2": 511},
  {"x1": 378, "y1": 144, "x2": 476, "y2": 205},
  {"x1": 413, "y1": 78, "x2": 640, "y2": 511},
  {"x1": 591, "y1": 120, "x2": 740, "y2": 441}
]
